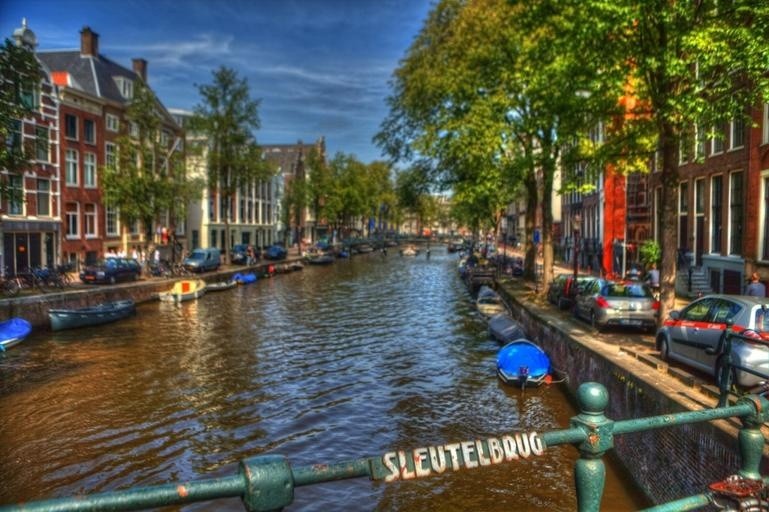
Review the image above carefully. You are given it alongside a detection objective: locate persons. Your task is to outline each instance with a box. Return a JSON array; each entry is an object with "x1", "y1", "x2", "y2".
[
  {"x1": 745, "y1": 272, "x2": 765, "y2": 331},
  {"x1": 155, "y1": 222, "x2": 162, "y2": 243},
  {"x1": 643, "y1": 262, "x2": 659, "y2": 299},
  {"x1": 103, "y1": 247, "x2": 146, "y2": 264},
  {"x1": 246, "y1": 244, "x2": 253, "y2": 266},
  {"x1": 161, "y1": 224, "x2": 168, "y2": 244}
]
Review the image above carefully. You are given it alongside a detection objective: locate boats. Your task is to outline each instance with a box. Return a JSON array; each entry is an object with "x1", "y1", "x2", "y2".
[
  {"x1": 457, "y1": 253, "x2": 552, "y2": 387},
  {"x1": 48, "y1": 299, "x2": 135, "y2": 332},
  {"x1": 157, "y1": 271, "x2": 257, "y2": 302},
  {"x1": 0, "y1": 317, "x2": 32, "y2": 352}
]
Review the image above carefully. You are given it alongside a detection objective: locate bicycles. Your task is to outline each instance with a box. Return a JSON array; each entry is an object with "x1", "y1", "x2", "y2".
[{"x1": 0, "y1": 265, "x2": 76, "y2": 297}]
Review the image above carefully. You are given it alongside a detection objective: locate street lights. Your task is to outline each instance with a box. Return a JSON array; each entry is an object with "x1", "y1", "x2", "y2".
[
  {"x1": 501, "y1": 209, "x2": 508, "y2": 273},
  {"x1": 572, "y1": 215, "x2": 582, "y2": 316}
]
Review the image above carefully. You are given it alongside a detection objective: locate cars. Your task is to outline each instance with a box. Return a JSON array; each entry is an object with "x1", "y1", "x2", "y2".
[
  {"x1": 547, "y1": 273, "x2": 660, "y2": 333},
  {"x1": 183, "y1": 245, "x2": 287, "y2": 274},
  {"x1": 656, "y1": 293, "x2": 769, "y2": 394},
  {"x1": 79, "y1": 256, "x2": 142, "y2": 284}
]
